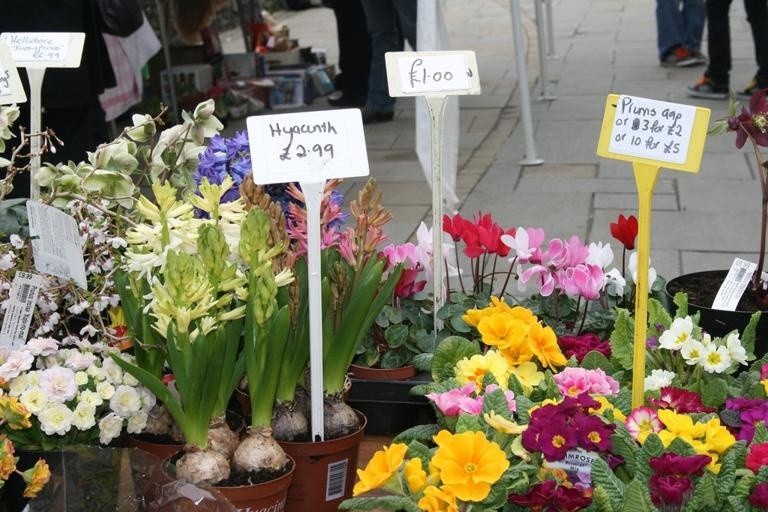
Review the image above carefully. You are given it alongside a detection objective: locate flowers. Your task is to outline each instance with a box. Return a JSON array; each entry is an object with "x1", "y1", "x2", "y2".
[{"x1": 0, "y1": 88, "x2": 768, "y2": 512}]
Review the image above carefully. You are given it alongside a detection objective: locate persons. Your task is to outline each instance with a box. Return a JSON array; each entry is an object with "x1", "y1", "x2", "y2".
[
  {"x1": 654, "y1": 0, "x2": 708, "y2": 67},
  {"x1": 321, "y1": 0, "x2": 406, "y2": 124},
  {"x1": 685, "y1": 0, "x2": 768, "y2": 100}
]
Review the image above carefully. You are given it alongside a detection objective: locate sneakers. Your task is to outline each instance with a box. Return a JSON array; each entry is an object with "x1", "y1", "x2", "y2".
[
  {"x1": 361, "y1": 105, "x2": 394, "y2": 123},
  {"x1": 735, "y1": 78, "x2": 768, "y2": 101},
  {"x1": 661, "y1": 46, "x2": 706, "y2": 66},
  {"x1": 687, "y1": 76, "x2": 730, "y2": 99},
  {"x1": 328, "y1": 93, "x2": 364, "y2": 107}
]
